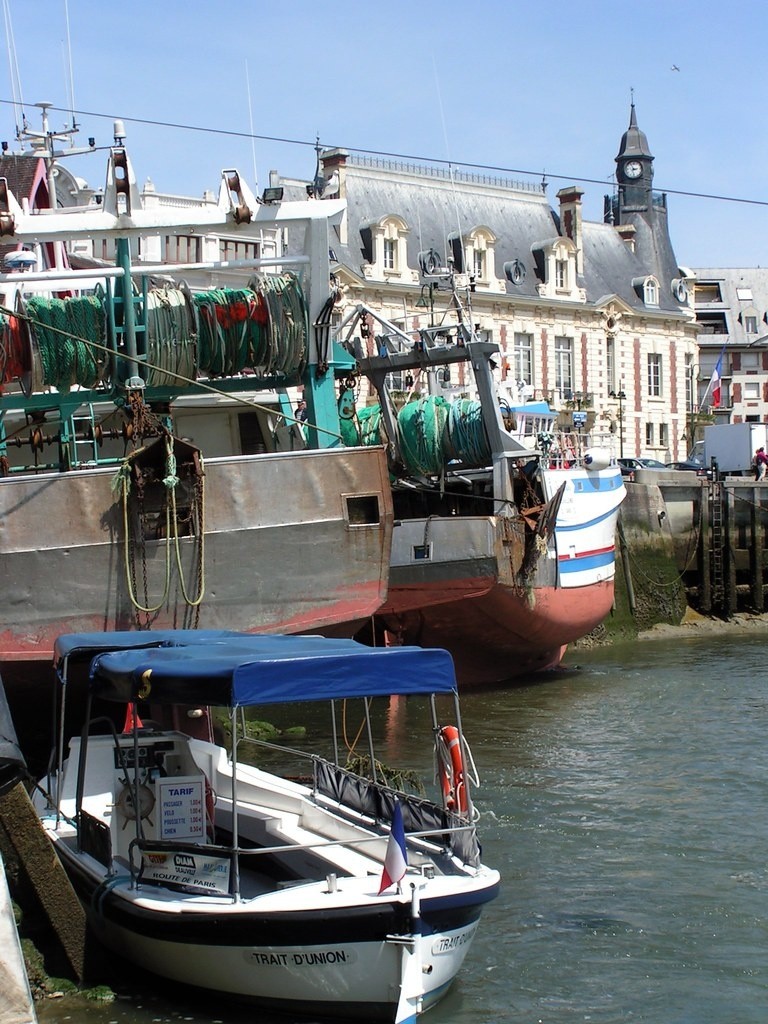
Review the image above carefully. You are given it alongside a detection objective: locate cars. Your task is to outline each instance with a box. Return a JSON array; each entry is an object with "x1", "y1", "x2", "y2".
[{"x1": 616, "y1": 456, "x2": 716, "y2": 482}]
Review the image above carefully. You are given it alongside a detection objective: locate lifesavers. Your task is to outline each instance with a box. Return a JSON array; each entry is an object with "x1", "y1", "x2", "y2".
[
  {"x1": 437, "y1": 725, "x2": 469, "y2": 819},
  {"x1": 199, "y1": 767, "x2": 215, "y2": 837}
]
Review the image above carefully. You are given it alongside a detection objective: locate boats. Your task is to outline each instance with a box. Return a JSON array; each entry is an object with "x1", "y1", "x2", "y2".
[
  {"x1": 1, "y1": 0, "x2": 628, "y2": 692},
  {"x1": 28, "y1": 624, "x2": 504, "y2": 1024}
]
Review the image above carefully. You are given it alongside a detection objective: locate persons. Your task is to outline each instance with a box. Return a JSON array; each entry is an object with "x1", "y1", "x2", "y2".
[{"x1": 753, "y1": 447, "x2": 768, "y2": 482}]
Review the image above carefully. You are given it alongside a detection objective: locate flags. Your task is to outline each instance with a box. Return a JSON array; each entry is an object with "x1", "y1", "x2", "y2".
[
  {"x1": 377, "y1": 800, "x2": 409, "y2": 895},
  {"x1": 712, "y1": 352, "x2": 723, "y2": 406}
]
